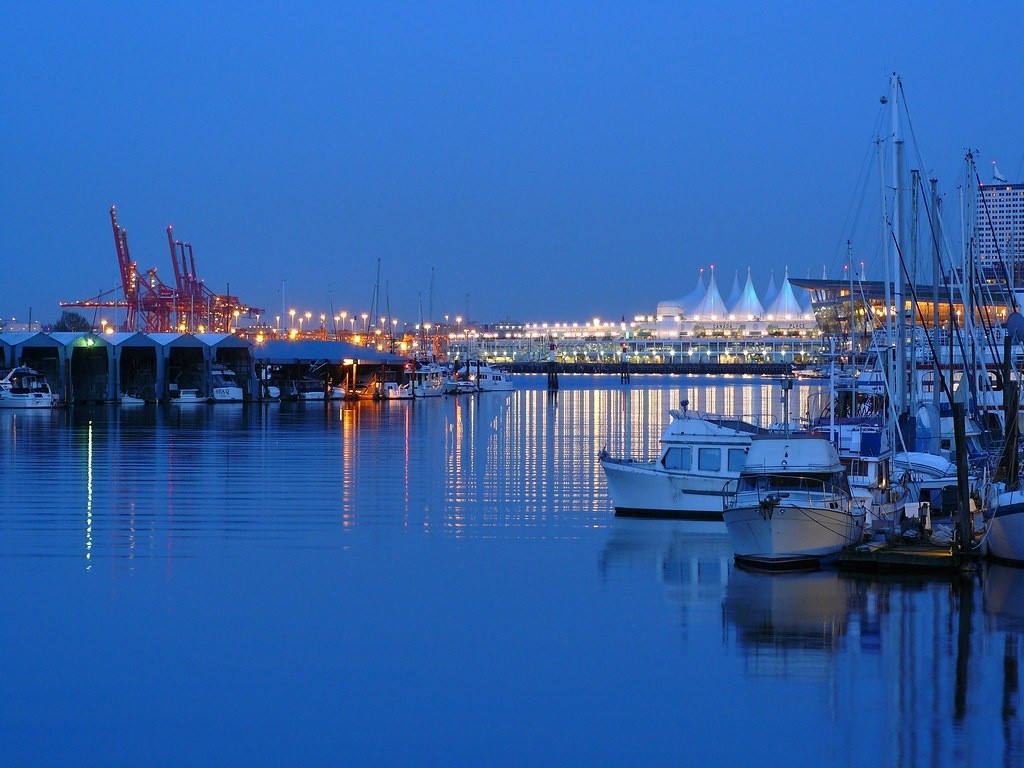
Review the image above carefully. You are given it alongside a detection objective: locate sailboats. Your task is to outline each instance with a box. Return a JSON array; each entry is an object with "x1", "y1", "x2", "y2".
[{"x1": 783, "y1": 62, "x2": 1023, "y2": 571}]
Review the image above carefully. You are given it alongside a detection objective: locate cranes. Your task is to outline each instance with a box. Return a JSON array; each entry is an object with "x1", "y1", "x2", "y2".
[{"x1": 58, "y1": 200, "x2": 266, "y2": 333}]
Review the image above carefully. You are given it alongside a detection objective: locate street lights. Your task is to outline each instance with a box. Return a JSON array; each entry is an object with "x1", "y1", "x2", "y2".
[
  {"x1": 455, "y1": 316, "x2": 462, "y2": 333},
  {"x1": 320, "y1": 314, "x2": 326, "y2": 330},
  {"x1": 305, "y1": 312, "x2": 312, "y2": 332},
  {"x1": 339, "y1": 311, "x2": 347, "y2": 331},
  {"x1": 288, "y1": 308, "x2": 296, "y2": 329},
  {"x1": 334, "y1": 316, "x2": 340, "y2": 335},
  {"x1": 348, "y1": 319, "x2": 355, "y2": 332},
  {"x1": 102, "y1": 320, "x2": 108, "y2": 332},
  {"x1": 380, "y1": 318, "x2": 386, "y2": 336},
  {"x1": 362, "y1": 314, "x2": 369, "y2": 332},
  {"x1": 424, "y1": 323, "x2": 432, "y2": 351},
  {"x1": 275, "y1": 315, "x2": 281, "y2": 339},
  {"x1": 234, "y1": 310, "x2": 240, "y2": 329},
  {"x1": 298, "y1": 318, "x2": 304, "y2": 331},
  {"x1": 392, "y1": 320, "x2": 397, "y2": 338},
  {"x1": 255, "y1": 314, "x2": 260, "y2": 333}
]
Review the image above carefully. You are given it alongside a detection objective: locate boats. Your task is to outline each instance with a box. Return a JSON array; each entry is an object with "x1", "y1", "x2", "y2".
[
  {"x1": 717, "y1": 334, "x2": 869, "y2": 574},
  {"x1": 0, "y1": 362, "x2": 59, "y2": 409},
  {"x1": 594, "y1": 397, "x2": 778, "y2": 523},
  {"x1": 210, "y1": 360, "x2": 518, "y2": 404},
  {"x1": 121, "y1": 387, "x2": 145, "y2": 403},
  {"x1": 169, "y1": 387, "x2": 214, "y2": 404}
]
